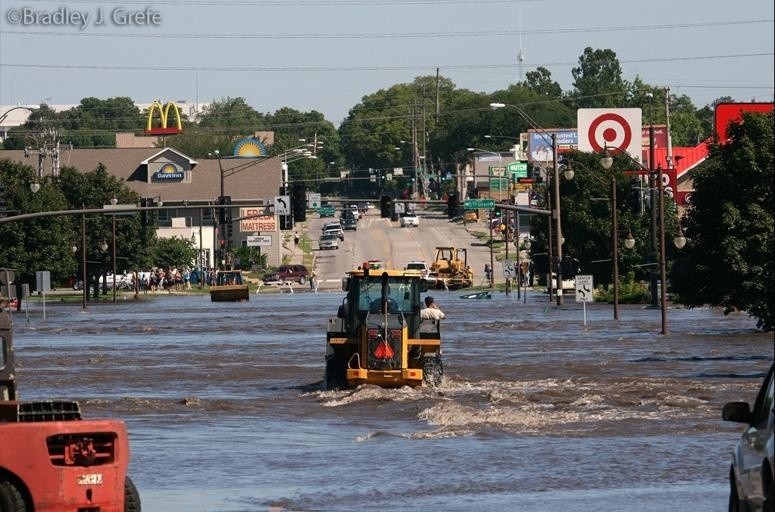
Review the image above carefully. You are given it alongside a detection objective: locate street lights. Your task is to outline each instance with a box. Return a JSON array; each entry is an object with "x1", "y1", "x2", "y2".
[
  {"x1": 112, "y1": 193, "x2": 163, "y2": 304},
  {"x1": 394, "y1": 139, "x2": 429, "y2": 197},
  {"x1": 467, "y1": 149, "x2": 503, "y2": 224},
  {"x1": 484, "y1": 135, "x2": 521, "y2": 141},
  {"x1": 0, "y1": 172, "x2": 40, "y2": 219},
  {"x1": 489, "y1": 103, "x2": 564, "y2": 306},
  {"x1": 80, "y1": 188, "x2": 118, "y2": 309},
  {"x1": 214, "y1": 138, "x2": 336, "y2": 270},
  {"x1": 599, "y1": 141, "x2": 687, "y2": 335},
  {"x1": 565, "y1": 158, "x2": 635, "y2": 321}
]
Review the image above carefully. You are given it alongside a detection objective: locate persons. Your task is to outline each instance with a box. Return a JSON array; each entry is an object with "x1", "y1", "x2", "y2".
[
  {"x1": 118, "y1": 265, "x2": 218, "y2": 295},
  {"x1": 531, "y1": 144, "x2": 554, "y2": 162},
  {"x1": 294, "y1": 231, "x2": 299, "y2": 244},
  {"x1": 421, "y1": 296, "x2": 446, "y2": 320},
  {"x1": 369, "y1": 285, "x2": 398, "y2": 311},
  {"x1": 484, "y1": 263, "x2": 493, "y2": 281}
]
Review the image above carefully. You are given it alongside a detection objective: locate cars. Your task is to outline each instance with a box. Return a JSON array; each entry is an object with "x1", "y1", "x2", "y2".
[
  {"x1": 381, "y1": 195, "x2": 420, "y2": 228},
  {"x1": 318, "y1": 183, "x2": 378, "y2": 249},
  {"x1": 722, "y1": 361, "x2": 775, "y2": 510},
  {"x1": 462, "y1": 211, "x2": 478, "y2": 223},
  {"x1": 92, "y1": 267, "x2": 152, "y2": 291}
]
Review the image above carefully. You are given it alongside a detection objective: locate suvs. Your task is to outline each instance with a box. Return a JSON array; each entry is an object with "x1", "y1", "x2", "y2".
[
  {"x1": 404, "y1": 260, "x2": 429, "y2": 283},
  {"x1": 261, "y1": 265, "x2": 308, "y2": 286}
]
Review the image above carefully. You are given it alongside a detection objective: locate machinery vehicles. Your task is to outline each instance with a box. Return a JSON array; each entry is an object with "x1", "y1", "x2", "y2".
[
  {"x1": 325, "y1": 262, "x2": 443, "y2": 391},
  {"x1": 427, "y1": 247, "x2": 473, "y2": 292},
  {"x1": 0, "y1": 306, "x2": 141, "y2": 511},
  {"x1": 209, "y1": 270, "x2": 249, "y2": 302}
]
